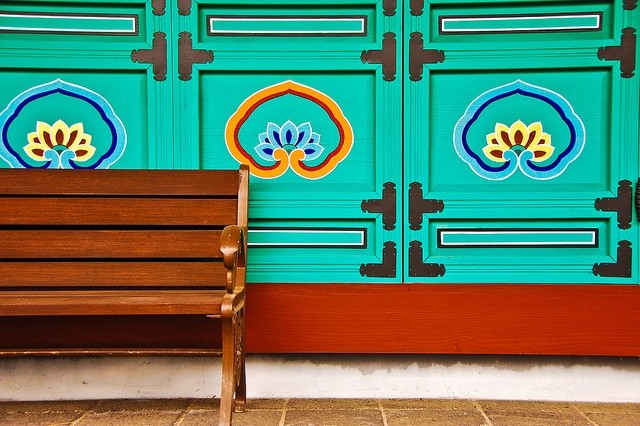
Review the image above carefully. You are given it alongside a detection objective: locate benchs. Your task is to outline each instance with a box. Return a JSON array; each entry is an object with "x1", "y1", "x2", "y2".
[{"x1": 0, "y1": 164, "x2": 249, "y2": 426}]
